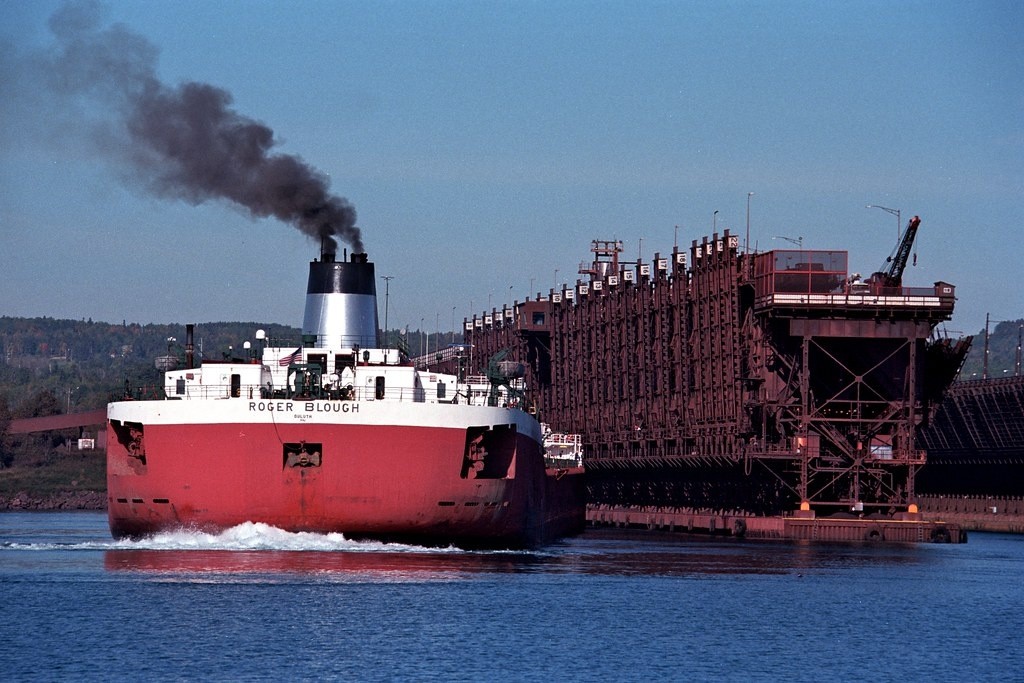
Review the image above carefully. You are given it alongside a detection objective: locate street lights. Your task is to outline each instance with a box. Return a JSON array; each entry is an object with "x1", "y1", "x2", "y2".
[
  {"x1": 380, "y1": 275, "x2": 394, "y2": 349},
  {"x1": 772, "y1": 236, "x2": 803, "y2": 263},
  {"x1": 420, "y1": 317, "x2": 425, "y2": 356},
  {"x1": 453, "y1": 306, "x2": 457, "y2": 343},
  {"x1": 747, "y1": 192, "x2": 756, "y2": 253},
  {"x1": 436, "y1": 313, "x2": 440, "y2": 352},
  {"x1": 865, "y1": 204, "x2": 901, "y2": 241},
  {"x1": 639, "y1": 237, "x2": 644, "y2": 259},
  {"x1": 714, "y1": 210, "x2": 719, "y2": 233},
  {"x1": 530, "y1": 278, "x2": 536, "y2": 300},
  {"x1": 509, "y1": 285, "x2": 513, "y2": 307},
  {"x1": 554, "y1": 269, "x2": 560, "y2": 293},
  {"x1": 674, "y1": 225, "x2": 679, "y2": 246}
]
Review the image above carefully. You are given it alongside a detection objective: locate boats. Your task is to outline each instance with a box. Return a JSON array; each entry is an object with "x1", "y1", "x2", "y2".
[{"x1": 107, "y1": 237, "x2": 589, "y2": 550}]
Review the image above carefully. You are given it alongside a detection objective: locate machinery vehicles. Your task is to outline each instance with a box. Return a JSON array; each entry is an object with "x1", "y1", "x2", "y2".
[{"x1": 864, "y1": 215, "x2": 921, "y2": 295}]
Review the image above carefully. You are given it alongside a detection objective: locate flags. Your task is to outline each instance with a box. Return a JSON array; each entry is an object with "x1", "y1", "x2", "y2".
[{"x1": 279, "y1": 346, "x2": 303, "y2": 366}]
